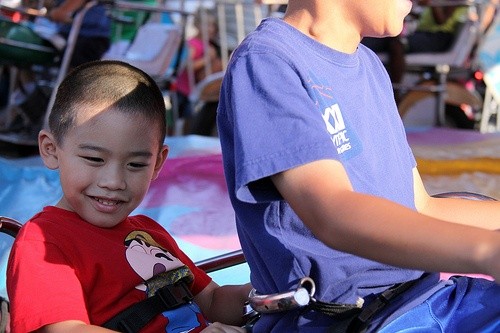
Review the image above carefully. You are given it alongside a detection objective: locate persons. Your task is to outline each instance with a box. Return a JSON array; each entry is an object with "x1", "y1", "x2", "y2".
[
  {"x1": 6, "y1": 60, "x2": 255, "y2": 333},
  {"x1": 215, "y1": 0, "x2": 500, "y2": 333},
  {"x1": 170, "y1": 7, "x2": 217, "y2": 136}
]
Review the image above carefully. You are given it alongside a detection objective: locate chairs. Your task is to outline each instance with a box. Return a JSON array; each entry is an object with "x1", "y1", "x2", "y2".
[{"x1": 377, "y1": 21, "x2": 478, "y2": 126}]
